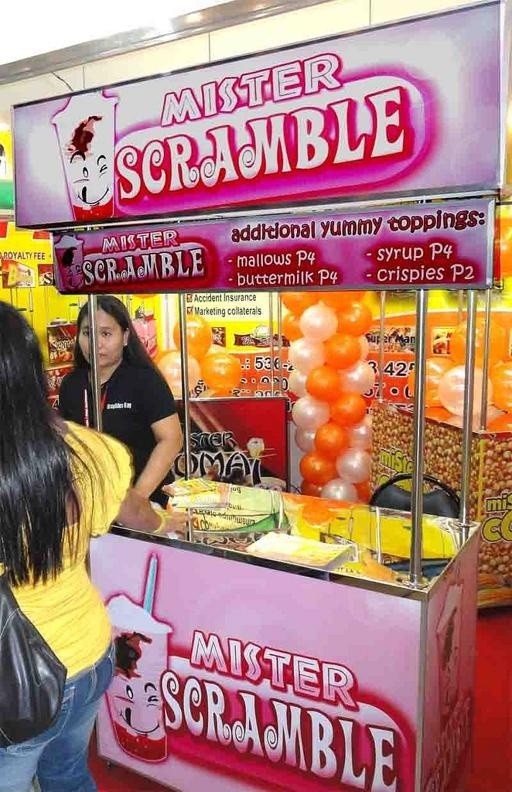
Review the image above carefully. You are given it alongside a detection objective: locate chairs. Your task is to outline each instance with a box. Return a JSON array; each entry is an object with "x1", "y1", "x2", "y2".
[{"x1": 366, "y1": 471, "x2": 462, "y2": 519}]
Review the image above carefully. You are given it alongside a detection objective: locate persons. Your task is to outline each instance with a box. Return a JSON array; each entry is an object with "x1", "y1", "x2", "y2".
[
  {"x1": 0, "y1": 301, "x2": 161, "y2": 791},
  {"x1": 60, "y1": 297, "x2": 184, "y2": 531}
]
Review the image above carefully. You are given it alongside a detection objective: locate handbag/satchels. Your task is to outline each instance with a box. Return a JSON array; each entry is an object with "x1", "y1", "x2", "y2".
[{"x1": 0, "y1": 573, "x2": 67, "y2": 748}]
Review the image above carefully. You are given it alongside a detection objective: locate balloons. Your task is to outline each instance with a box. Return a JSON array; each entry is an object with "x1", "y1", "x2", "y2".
[
  {"x1": 280, "y1": 292, "x2": 381, "y2": 503},
  {"x1": 494, "y1": 210, "x2": 512, "y2": 279},
  {"x1": 407, "y1": 316, "x2": 512, "y2": 433},
  {"x1": 158, "y1": 314, "x2": 241, "y2": 399}
]
